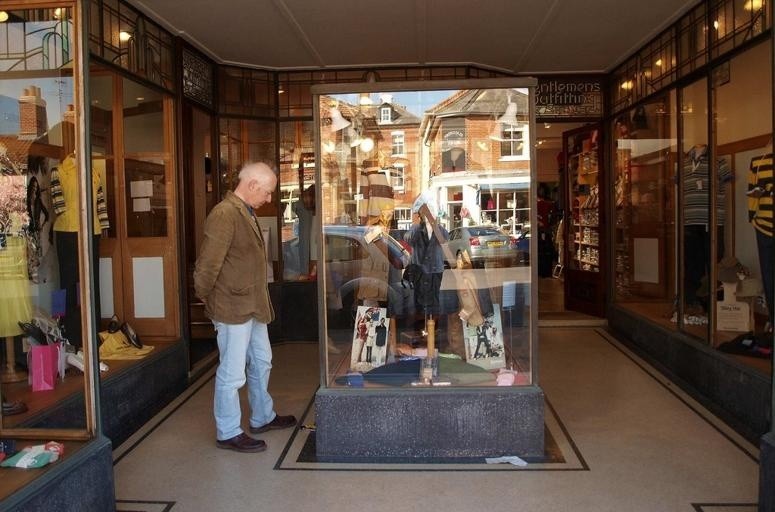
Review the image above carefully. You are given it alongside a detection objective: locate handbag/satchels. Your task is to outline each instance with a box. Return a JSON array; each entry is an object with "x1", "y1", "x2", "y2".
[
  {"x1": 31, "y1": 340, "x2": 59, "y2": 393},
  {"x1": 402, "y1": 263, "x2": 423, "y2": 284}
]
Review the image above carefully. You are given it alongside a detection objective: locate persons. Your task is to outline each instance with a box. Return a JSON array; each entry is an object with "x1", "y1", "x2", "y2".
[
  {"x1": 747, "y1": 139, "x2": 774, "y2": 328},
  {"x1": 27, "y1": 176, "x2": 49, "y2": 254},
  {"x1": 358, "y1": 317, "x2": 386, "y2": 366},
  {"x1": 429, "y1": 147, "x2": 493, "y2": 318},
  {"x1": 403, "y1": 208, "x2": 449, "y2": 336},
  {"x1": 193, "y1": 161, "x2": 297, "y2": 453},
  {"x1": 673, "y1": 143, "x2": 731, "y2": 315},
  {"x1": 359, "y1": 143, "x2": 413, "y2": 307},
  {"x1": 50, "y1": 150, "x2": 110, "y2": 346},
  {"x1": 473, "y1": 321, "x2": 497, "y2": 360}
]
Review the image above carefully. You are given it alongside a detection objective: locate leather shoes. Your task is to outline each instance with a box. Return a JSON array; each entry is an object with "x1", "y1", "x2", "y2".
[
  {"x1": 217, "y1": 434, "x2": 266, "y2": 453},
  {"x1": 121, "y1": 321, "x2": 143, "y2": 349},
  {"x1": 67, "y1": 354, "x2": 85, "y2": 373},
  {"x1": 3, "y1": 399, "x2": 28, "y2": 415},
  {"x1": 108, "y1": 314, "x2": 120, "y2": 333},
  {"x1": 250, "y1": 414, "x2": 297, "y2": 434},
  {"x1": 78, "y1": 350, "x2": 109, "y2": 372}
]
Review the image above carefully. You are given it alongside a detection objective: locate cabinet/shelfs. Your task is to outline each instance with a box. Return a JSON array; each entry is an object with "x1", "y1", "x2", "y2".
[{"x1": 562, "y1": 123, "x2": 631, "y2": 318}]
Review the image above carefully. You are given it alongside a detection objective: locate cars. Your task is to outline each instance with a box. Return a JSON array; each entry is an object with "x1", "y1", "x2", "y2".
[
  {"x1": 441, "y1": 223, "x2": 530, "y2": 271},
  {"x1": 282, "y1": 223, "x2": 414, "y2": 331}
]
